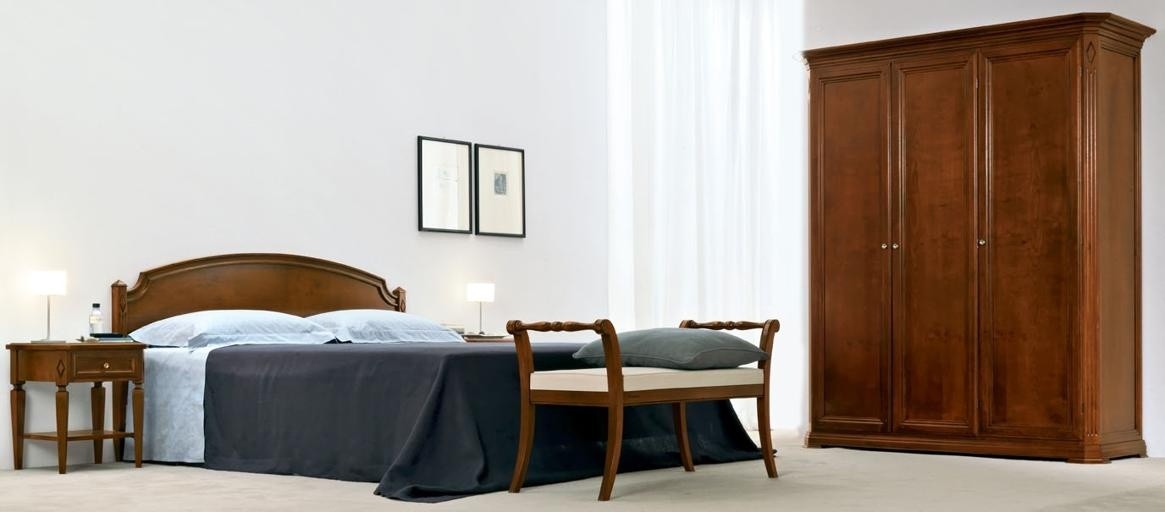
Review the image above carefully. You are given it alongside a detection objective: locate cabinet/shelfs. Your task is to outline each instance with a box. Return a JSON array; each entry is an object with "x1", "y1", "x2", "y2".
[{"x1": 803, "y1": 11, "x2": 1159, "y2": 465}]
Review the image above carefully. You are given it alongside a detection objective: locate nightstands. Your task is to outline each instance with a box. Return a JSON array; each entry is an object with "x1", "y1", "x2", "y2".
[
  {"x1": 461, "y1": 334, "x2": 513, "y2": 343},
  {"x1": 5, "y1": 342, "x2": 147, "y2": 473}
]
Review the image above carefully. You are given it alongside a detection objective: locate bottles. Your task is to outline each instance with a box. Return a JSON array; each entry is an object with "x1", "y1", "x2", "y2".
[{"x1": 88, "y1": 303, "x2": 104, "y2": 334}]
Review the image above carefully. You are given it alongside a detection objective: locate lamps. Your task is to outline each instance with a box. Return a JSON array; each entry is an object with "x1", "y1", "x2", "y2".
[
  {"x1": 26, "y1": 269, "x2": 71, "y2": 345},
  {"x1": 464, "y1": 267, "x2": 497, "y2": 335}
]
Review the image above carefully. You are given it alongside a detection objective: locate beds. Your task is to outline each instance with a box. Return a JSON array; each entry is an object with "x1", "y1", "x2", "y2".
[{"x1": 110, "y1": 252, "x2": 778, "y2": 503}]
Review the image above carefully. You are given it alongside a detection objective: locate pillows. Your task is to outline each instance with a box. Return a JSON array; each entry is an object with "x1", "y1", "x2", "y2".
[
  {"x1": 128, "y1": 310, "x2": 335, "y2": 348},
  {"x1": 571, "y1": 326, "x2": 772, "y2": 371},
  {"x1": 305, "y1": 309, "x2": 467, "y2": 344}
]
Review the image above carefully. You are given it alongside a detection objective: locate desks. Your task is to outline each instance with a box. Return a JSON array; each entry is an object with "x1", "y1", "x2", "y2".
[{"x1": 505, "y1": 317, "x2": 780, "y2": 500}]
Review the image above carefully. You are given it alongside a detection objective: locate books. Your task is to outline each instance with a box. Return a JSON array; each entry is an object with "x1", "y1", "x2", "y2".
[{"x1": 96, "y1": 336, "x2": 135, "y2": 343}]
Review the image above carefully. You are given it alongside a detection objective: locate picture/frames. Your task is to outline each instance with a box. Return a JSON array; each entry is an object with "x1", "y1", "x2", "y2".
[{"x1": 416, "y1": 135, "x2": 527, "y2": 240}]
